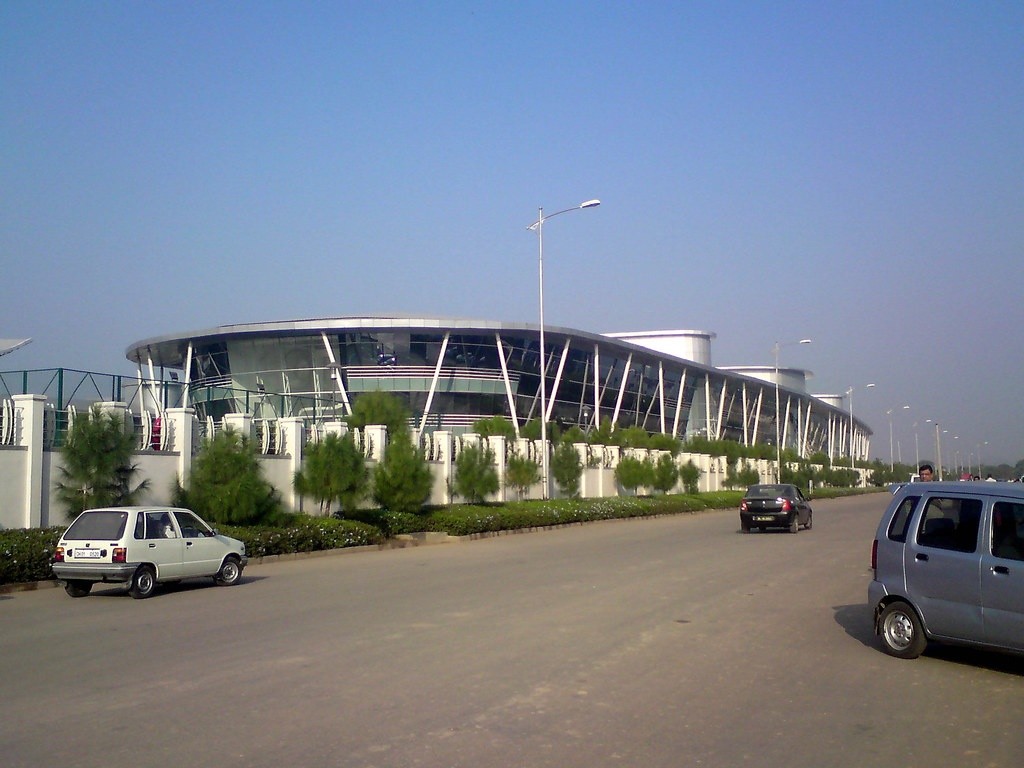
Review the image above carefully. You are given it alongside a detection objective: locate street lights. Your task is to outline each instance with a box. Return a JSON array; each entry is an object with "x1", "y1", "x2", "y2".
[
  {"x1": 525, "y1": 200, "x2": 602, "y2": 499},
  {"x1": 844, "y1": 383, "x2": 877, "y2": 488},
  {"x1": 887, "y1": 405, "x2": 910, "y2": 487},
  {"x1": 911, "y1": 418, "x2": 991, "y2": 484},
  {"x1": 771, "y1": 338, "x2": 810, "y2": 484}
]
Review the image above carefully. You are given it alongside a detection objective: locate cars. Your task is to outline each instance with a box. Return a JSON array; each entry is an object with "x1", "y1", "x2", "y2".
[
  {"x1": 739, "y1": 484, "x2": 813, "y2": 533},
  {"x1": 49, "y1": 505, "x2": 249, "y2": 600}
]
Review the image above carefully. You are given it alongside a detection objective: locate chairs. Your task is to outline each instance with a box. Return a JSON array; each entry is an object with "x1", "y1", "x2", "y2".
[{"x1": 925, "y1": 517, "x2": 957, "y2": 550}]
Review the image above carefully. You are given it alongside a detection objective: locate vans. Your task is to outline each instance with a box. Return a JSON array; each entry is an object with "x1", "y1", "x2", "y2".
[{"x1": 864, "y1": 480, "x2": 1024, "y2": 662}]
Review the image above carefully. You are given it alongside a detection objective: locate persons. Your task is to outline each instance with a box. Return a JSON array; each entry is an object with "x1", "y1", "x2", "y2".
[
  {"x1": 161, "y1": 514, "x2": 176, "y2": 538},
  {"x1": 911, "y1": 465, "x2": 945, "y2": 530},
  {"x1": 966, "y1": 473, "x2": 1022, "y2": 483}
]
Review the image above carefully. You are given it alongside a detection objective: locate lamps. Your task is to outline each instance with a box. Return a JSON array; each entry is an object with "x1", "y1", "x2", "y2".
[
  {"x1": 581, "y1": 406, "x2": 591, "y2": 417},
  {"x1": 327, "y1": 363, "x2": 342, "y2": 380}
]
